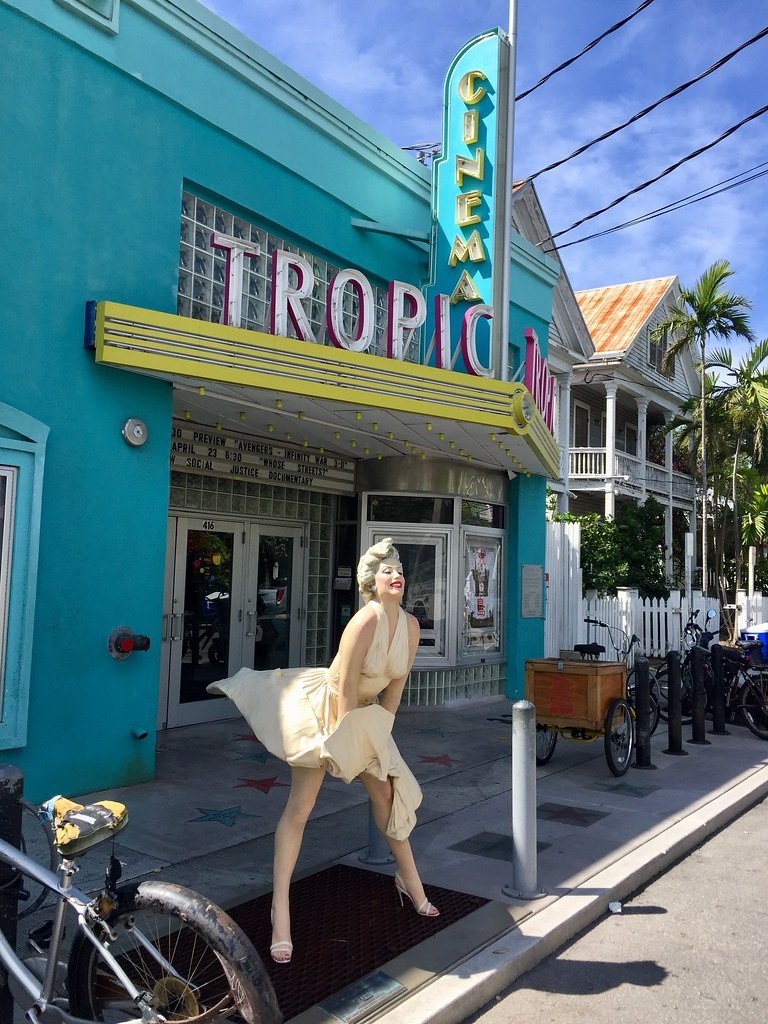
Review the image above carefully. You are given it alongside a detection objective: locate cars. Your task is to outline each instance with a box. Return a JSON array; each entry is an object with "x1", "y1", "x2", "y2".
[{"x1": 257, "y1": 576, "x2": 290, "y2": 610}]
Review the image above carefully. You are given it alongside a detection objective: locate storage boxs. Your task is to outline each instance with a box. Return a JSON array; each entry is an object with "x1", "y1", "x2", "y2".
[{"x1": 739, "y1": 621, "x2": 768, "y2": 661}]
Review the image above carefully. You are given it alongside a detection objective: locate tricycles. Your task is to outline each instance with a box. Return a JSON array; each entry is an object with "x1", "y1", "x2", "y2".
[{"x1": 526, "y1": 616, "x2": 662, "y2": 775}]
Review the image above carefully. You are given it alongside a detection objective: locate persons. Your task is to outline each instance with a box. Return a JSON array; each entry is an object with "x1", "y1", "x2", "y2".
[
  {"x1": 206, "y1": 537, "x2": 440, "y2": 964},
  {"x1": 413, "y1": 601, "x2": 433, "y2": 629},
  {"x1": 182, "y1": 579, "x2": 210, "y2": 670}
]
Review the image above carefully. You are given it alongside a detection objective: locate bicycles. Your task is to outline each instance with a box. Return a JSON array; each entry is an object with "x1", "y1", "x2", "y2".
[
  {"x1": 626, "y1": 609, "x2": 768, "y2": 741},
  {"x1": 0, "y1": 794, "x2": 281, "y2": 1024}
]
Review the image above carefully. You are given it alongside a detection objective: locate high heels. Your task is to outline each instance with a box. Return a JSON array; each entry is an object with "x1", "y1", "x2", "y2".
[
  {"x1": 395, "y1": 870, "x2": 438, "y2": 916},
  {"x1": 270, "y1": 911, "x2": 293, "y2": 964}
]
